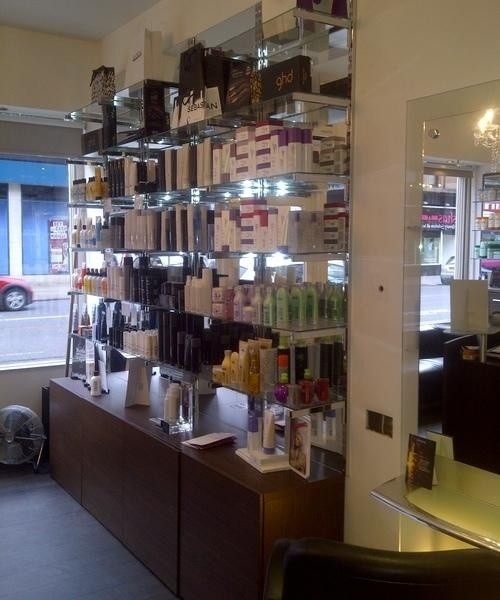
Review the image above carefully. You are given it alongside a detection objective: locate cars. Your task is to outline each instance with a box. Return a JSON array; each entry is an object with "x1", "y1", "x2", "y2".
[
  {"x1": 439, "y1": 255, "x2": 455, "y2": 285},
  {"x1": 0, "y1": 275, "x2": 35, "y2": 311}
]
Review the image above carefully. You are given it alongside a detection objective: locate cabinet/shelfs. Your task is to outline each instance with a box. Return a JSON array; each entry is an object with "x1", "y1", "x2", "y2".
[{"x1": 45, "y1": 58, "x2": 356, "y2": 600}]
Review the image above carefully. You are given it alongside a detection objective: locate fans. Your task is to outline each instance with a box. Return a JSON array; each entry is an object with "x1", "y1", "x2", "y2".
[{"x1": 0, "y1": 405, "x2": 48, "y2": 476}]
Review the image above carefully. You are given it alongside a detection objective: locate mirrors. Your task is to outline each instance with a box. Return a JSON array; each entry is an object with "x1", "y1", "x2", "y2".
[{"x1": 402, "y1": 78, "x2": 500, "y2": 537}]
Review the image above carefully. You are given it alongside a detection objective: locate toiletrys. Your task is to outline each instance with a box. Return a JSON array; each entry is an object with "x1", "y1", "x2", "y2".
[{"x1": 71, "y1": 119, "x2": 352, "y2": 454}]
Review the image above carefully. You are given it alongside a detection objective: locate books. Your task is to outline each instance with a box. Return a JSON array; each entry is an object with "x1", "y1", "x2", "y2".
[
  {"x1": 180, "y1": 430, "x2": 238, "y2": 450},
  {"x1": 235, "y1": 445, "x2": 291, "y2": 474}
]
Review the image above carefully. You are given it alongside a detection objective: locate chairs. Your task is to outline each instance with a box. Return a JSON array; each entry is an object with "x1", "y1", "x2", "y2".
[{"x1": 419, "y1": 327, "x2": 476, "y2": 426}]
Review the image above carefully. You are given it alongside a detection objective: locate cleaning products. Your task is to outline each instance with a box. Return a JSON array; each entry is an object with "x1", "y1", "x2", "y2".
[{"x1": 474, "y1": 202, "x2": 500, "y2": 258}]
